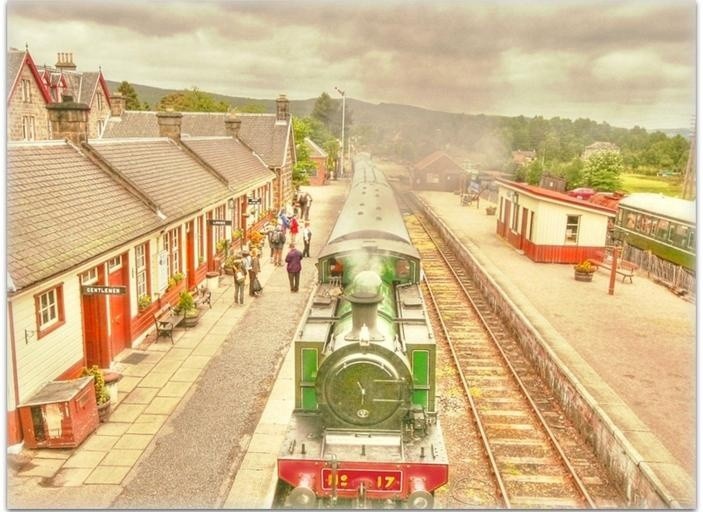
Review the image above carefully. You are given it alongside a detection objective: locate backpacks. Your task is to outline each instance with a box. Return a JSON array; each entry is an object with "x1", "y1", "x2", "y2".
[
  {"x1": 271, "y1": 231, "x2": 280, "y2": 244},
  {"x1": 234, "y1": 265, "x2": 246, "y2": 284}
]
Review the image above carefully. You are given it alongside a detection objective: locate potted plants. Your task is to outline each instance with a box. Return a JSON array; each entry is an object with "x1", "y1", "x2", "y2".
[
  {"x1": 573, "y1": 260, "x2": 594, "y2": 282},
  {"x1": 78, "y1": 365, "x2": 111, "y2": 424},
  {"x1": 175, "y1": 289, "x2": 200, "y2": 327}
]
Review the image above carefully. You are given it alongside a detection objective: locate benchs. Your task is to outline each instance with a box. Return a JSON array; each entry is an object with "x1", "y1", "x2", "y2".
[
  {"x1": 151, "y1": 303, "x2": 185, "y2": 345},
  {"x1": 190, "y1": 285, "x2": 211, "y2": 311},
  {"x1": 586, "y1": 250, "x2": 635, "y2": 283}
]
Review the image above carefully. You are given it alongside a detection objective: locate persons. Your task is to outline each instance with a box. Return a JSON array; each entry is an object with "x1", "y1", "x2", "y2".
[{"x1": 232, "y1": 192, "x2": 312, "y2": 305}]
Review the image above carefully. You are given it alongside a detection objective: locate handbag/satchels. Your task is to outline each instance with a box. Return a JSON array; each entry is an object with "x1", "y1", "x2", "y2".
[{"x1": 254, "y1": 278, "x2": 261, "y2": 291}]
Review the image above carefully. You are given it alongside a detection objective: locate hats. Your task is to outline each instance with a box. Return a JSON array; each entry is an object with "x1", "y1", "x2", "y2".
[{"x1": 249, "y1": 248, "x2": 261, "y2": 256}]
